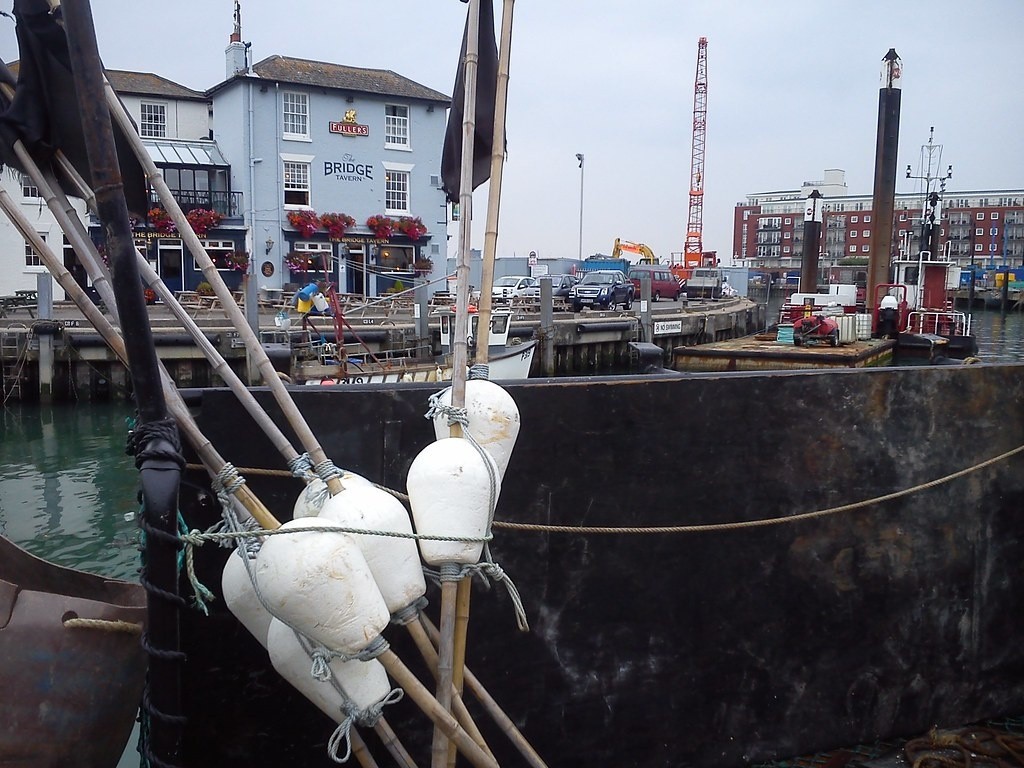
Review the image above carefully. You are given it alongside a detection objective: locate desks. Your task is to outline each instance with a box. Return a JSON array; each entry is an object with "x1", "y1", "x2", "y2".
[
  {"x1": 335, "y1": 293, "x2": 414, "y2": 317},
  {"x1": 174, "y1": 290, "x2": 228, "y2": 319},
  {"x1": 427, "y1": 292, "x2": 457, "y2": 317},
  {"x1": 233, "y1": 291, "x2": 266, "y2": 315},
  {"x1": 0, "y1": 290, "x2": 38, "y2": 319},
  {"x1": 98, "y1": 299, "x2": 108, "y2": 315},
  {"x1": 265, "y1": 289, "x2": 296, "y2": 314},
  {"x1": 492, "y1": 295, "x2": 565, "y2": 315}
]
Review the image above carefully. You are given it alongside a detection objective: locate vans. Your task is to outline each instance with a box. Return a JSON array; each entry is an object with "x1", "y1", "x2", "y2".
[{"x1": 627, "y1": 269, "x2": 681, "y2": 302}]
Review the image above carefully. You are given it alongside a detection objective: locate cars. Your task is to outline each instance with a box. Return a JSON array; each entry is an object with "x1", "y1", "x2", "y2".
[
  {"x1": 522, "y1": 274, "x2": 581, "y2": 310},
  {"x1": 491, "y1": 275, "x2": 538, "y2": 304}
]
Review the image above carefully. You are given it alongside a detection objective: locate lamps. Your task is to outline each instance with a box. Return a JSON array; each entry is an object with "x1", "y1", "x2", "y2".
[
  {"x1": 342, "y1": 243, "x2": 350, "y2": 259},
  {"x1": 372, "y1": 245, "x2": 378, "y2": 260},
  {"x1": 266, "y1": 237, "x2": 274, "y2": 255}
]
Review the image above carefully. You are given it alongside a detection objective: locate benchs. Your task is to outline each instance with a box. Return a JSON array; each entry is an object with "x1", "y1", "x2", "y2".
[
  {"x1": 270, "y1": 299, "x2": 285, "y2": 303},
  {"x1": 367, "y1": 305, "x2": 388, "y2": 309},
  {"x1": 402, "y1": 303, "x2": 414, "y2": 305},
  {"x1": 351, "y1": 304, "x2": 364, "y2": 307},
  {"x1": 495, "y1": 303, "x2": 573, "y2": 309},
  {"x1": 272, "y1": 305, "x2": 295, "y2": 309},
  {"x1": 338, "y1": 302, "x2": 352, "y2": 306},
  {"x1": 186, "y1": 305, "x2": 207, "y2": 309},
  {"x1": 428, "y1": 305, "x2": 442, "y2": 307},
  {"x1": 180, "y1": 301, "x2": 208, "y2": 305},
  {"x1": 6, "y1": 304, "x2": 37, "y2": 309},
  {"x1": 388, "y1": 307, "x2": 413, "y2": 311},
  {"x1": 237, "y1": 302, "x2": 270, "y2": 305},
  {"x1": 261, "y1": 299, "x2": 269, "y2": 302}
]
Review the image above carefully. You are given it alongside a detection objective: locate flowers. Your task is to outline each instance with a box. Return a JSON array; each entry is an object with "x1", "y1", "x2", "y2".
[
  {"x1": 224, "y1": 251, "x2": 250, "y2": 273},
  {"x1": 98, "y1": 243, "x2": 109, "y2": 269},
  {"x1": 367, "y1": 215, "x2": 428, "y2": 240},
  {"x1": 284, "y1": 251, "x2": 307, "y2": 274},
  {"x1": 128, "y1": 209, "x2": 177, "y2": 234},
  {"x1": 144, "y1": 289, "x2": 156, "y2": 305},
  {"x1": 286, "y1": 210, "x2": 357, "y2": 238},
  {"x1": 186, "y1": 208, "x2": 224, "y2": 235}
]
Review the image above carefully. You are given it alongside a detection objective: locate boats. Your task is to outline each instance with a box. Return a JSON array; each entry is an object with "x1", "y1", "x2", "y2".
[{"x1": 289, "y1": 327, "x2": 539, "y2": 388}]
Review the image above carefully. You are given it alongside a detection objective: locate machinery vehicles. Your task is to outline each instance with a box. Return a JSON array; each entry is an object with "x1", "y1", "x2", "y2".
[
  {"x1": 610, "y1": 237, "x2": 667, "y2": 280},
  {"x1": 672, "y1": 35, "x2": 722, "y2": 293}
]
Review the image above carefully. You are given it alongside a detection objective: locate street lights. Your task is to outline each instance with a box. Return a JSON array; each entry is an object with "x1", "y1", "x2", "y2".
[{"x1": 576, "y1": 153, "x2": 585, "y2": 260}]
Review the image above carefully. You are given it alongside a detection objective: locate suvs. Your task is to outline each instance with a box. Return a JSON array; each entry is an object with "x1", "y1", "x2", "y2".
[{"x1": 568, "y1": 269, "x2": 637, "y2": 312}]
